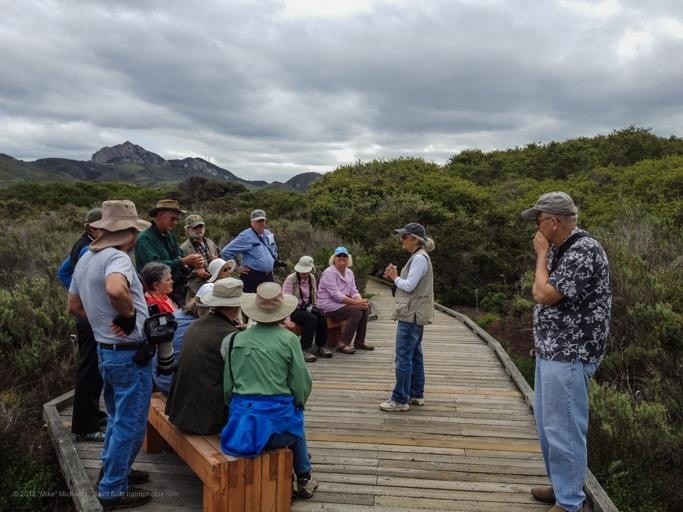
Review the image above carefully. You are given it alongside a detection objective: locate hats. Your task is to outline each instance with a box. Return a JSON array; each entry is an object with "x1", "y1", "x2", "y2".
[
  {"x1": 206, "y1": 258, "x2": 236, "y2": 283},
  {"x1": 250, "y1": 209, "x2": 267, "y2": 222},
  {"x1": 394, "y1": 222, "x2": 426, "y2": 241},
  {"x1": 82, "y1": 198, "x2": 206, "y2": 234},
  {"x1": 240, "y1": 281, "x2": 300, "y2": 324},
  {"x1": 520, "y1": 191, "x2": 578, "y2": 222},
  {"x1": 294, "y1": 255, "x2": 315, "y2": 274},
  {"x1": 333, "y1": 246, "x2": 349, "y2": 257},
  {"x1": 199, "y1": 276, "x2": 244, "y2": 309}
]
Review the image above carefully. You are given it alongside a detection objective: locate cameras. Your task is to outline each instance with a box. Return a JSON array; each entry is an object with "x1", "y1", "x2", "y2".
[
  {"x1": 144, "y1": 312, "x2": 178, "y2": 377},
  {"x1": 275, "y1": 260, "x2": 287, "y2": 271}
]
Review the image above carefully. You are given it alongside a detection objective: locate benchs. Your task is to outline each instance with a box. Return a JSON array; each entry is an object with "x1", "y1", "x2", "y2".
[
  {"x1": 288, "y1": 313, "x2": 351, "y2": 347},
  {"x1": 141, "y1": 391, "x2": 294, "y2": 509}
]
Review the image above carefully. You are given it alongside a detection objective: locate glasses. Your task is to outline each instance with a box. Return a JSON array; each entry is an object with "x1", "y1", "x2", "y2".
[
  {"x1": 400, "y1": 234, "x2": 411, "y2": 241},
  {"x1": 224, "y1": 267, "x2": 232, "y2": 273},
  {"x1": 336, "y1": 254, "x2": 348, "y2": 258},
  {"x1": 534, "y1": 216, "x2": 562, "y2": 227}
]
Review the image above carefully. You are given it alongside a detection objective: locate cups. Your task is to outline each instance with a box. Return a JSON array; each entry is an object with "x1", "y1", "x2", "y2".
[{"x1": 382, "y1": 262, "x2": 397, "y2": 280}]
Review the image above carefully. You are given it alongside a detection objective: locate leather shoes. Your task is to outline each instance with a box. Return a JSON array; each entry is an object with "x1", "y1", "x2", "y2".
[
  {"x1": 531, "y1": 484, "x2": 586, "y2": 506},
  {"x1": 547, "y1": 503, "x2": 584, "y2": 512}
]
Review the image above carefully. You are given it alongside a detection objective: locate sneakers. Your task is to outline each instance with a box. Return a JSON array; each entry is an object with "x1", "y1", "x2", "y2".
[
  {"x1": 303, "y1": 341, "x2": 374, "y2": 362},
  {"x1": 95, "y1": 465, "x2": 150, "y2": 489},
  {"x1": 408, "y1": 395, "x2": 425, "y2": 407},
  {"x1": 296, "y1": 473, "x2": 318, "y2": 499},
  {"x1": 75, "y1": 430, "x2": 106, "y2": 442},
  {"x1": 96, "y1": 486, "x2": 153, "y2": 512},
  {"x1": 98, "y1": 410, "x2": 109, "y2": 426},
  {"x1": 379, "y1": 398, "x2": 410, "y2": 413}
]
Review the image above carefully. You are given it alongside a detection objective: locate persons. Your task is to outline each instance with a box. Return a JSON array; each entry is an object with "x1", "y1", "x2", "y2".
[
  {"x1": 379, "y1": 223, "x2": 435, "y2": 413},
  {"x1": 521, "y1": 191, "x2": 612, "y2": 511}
]
